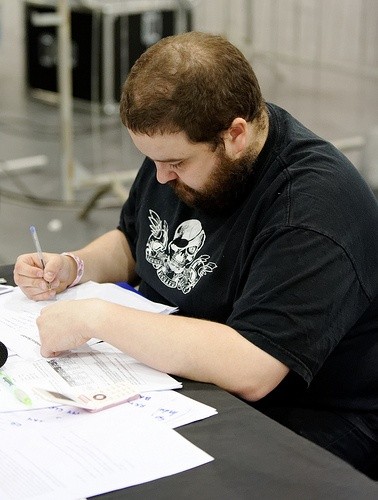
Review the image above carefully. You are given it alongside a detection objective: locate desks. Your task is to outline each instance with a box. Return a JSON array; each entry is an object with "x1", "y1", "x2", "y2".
[{"x1": 0, "y1": 259, "x2": 378, "y2": 499}]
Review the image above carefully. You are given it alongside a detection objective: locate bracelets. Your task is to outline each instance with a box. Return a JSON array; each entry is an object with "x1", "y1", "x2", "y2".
[{"x1": 62, "y1": 251, "x2": 83, "y2": 291}]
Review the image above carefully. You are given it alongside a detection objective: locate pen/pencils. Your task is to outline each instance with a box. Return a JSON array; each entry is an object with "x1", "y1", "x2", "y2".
[
  {"x1": 29, "y1": 226, "x2": 52, "y2": 291},
  {"x1": 0, "y1": 370, "x2": 32, "y2": 406}
]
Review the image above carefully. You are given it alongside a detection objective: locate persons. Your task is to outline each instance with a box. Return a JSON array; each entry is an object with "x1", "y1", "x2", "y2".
[{"x1": 8, "y1": 29, "x2": 376, "y2": 476}]
